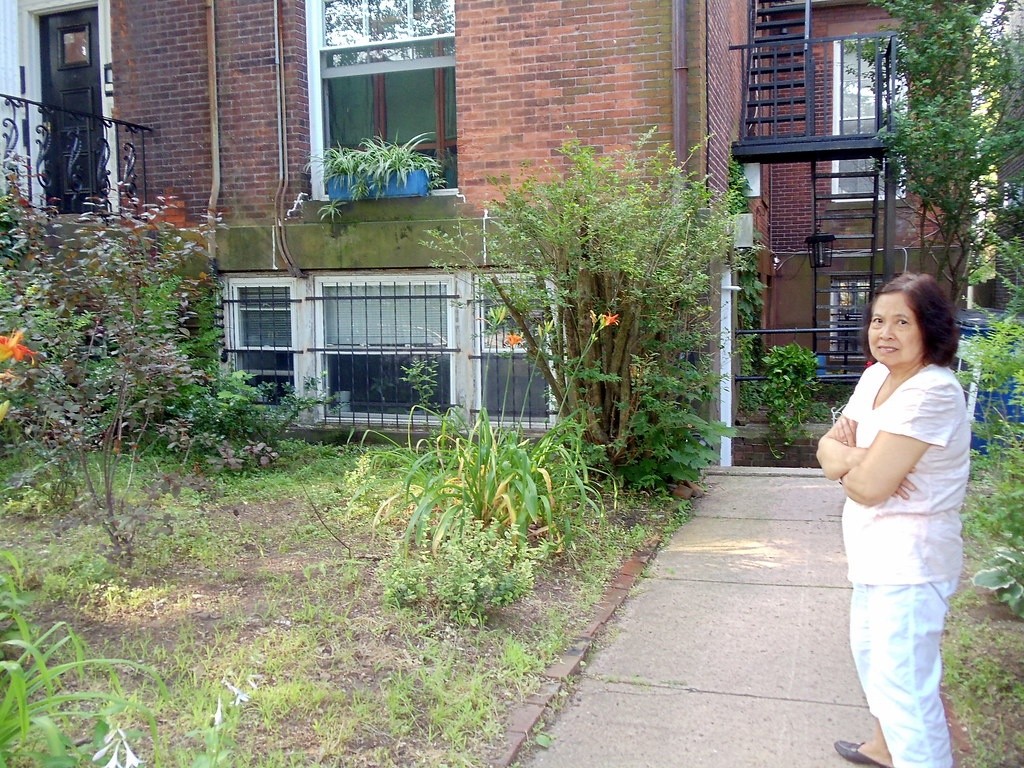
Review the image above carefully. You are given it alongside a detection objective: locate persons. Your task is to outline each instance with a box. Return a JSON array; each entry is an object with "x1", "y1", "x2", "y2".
[{"x1": 814, "y1": 271, "x2": 972, "y2": 768}]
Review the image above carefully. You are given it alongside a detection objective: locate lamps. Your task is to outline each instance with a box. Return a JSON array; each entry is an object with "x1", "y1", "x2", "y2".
[{"x1": 805, "y1": 216, "x2": 836, "y2": 269}]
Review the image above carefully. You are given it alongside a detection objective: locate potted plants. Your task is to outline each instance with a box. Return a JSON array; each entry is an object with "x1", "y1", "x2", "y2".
[{"x1": 301, "y1": 126, "x2": 449, "y2": 222}]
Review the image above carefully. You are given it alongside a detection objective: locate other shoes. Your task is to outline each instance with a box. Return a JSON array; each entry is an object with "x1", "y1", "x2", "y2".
[{"x1": 836, "y1": 741, "x2": 885, "y2": 767}]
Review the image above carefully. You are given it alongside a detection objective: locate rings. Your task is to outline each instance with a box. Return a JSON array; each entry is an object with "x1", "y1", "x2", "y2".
[{"x1": 842, "y1": 441, "x2": 848, "y2": 444}]
